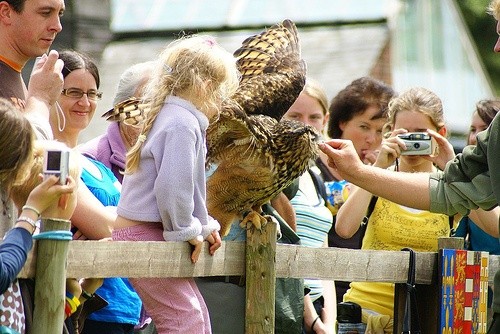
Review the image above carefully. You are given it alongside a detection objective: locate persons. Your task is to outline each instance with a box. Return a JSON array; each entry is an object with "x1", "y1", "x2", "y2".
[
  {"x1": 73, "y1": 61, "x2": 160, "y2": 183},
  {"x1": 282, "y1": 86, "x2": 337, "y2": 334},
  {"x1": 1, "y1": 1, "x2": 66, "y2": 111},
  {"x1": 0, "y1": 49, "x2": 65, "y2": 334},
  {"x1": 327, "y1": 75, "x2": 399, "y2": 302},
  {"x1": 317, "y1": 0, "x2": 500, "y2": 217},
  {"x1": 112, "y1": 34, "x2": 239, "y2": 334},
  {"x1": 335, "y1": 88, "x2": 499, "y2": 333},
  {"x1": 453, "y1": 98, "x2": 499, "y2": 254},
  {"x1": 52, "y1": 51, "x2": 142, "y2": 334},
  {"x1": 1, "y1": 98, "x2": 76, "y2": 296}
]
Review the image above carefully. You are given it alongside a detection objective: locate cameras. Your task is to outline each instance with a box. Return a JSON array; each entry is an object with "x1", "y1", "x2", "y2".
[
  {"x1": 396, "y1": 132, "x2": 432, "y2": 155},
  {"x1": 42, "y1": 149, "x2": 70, "y2": 186}
]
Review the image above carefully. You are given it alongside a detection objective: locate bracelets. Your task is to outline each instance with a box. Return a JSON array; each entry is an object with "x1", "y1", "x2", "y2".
[
  {"x1": 310, "y1": 315, "x2": 320, "y2": 332},
  {"x1": 22, "y1": 205, "x2": 41, "y2": 218},
  {"x1": 16, "y1": 217, "x2": 36, "y2": 229}
]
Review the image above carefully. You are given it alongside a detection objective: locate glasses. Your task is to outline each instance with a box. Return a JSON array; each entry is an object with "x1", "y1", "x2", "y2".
[{"x1": 61, "y1": 88, "x2": 103, "y2": 101}]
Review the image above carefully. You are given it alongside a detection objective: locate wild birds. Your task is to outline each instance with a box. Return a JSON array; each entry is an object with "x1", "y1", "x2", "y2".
[{"x1": 102, "y1": 19, "x2": 322, "y2": 233}]
